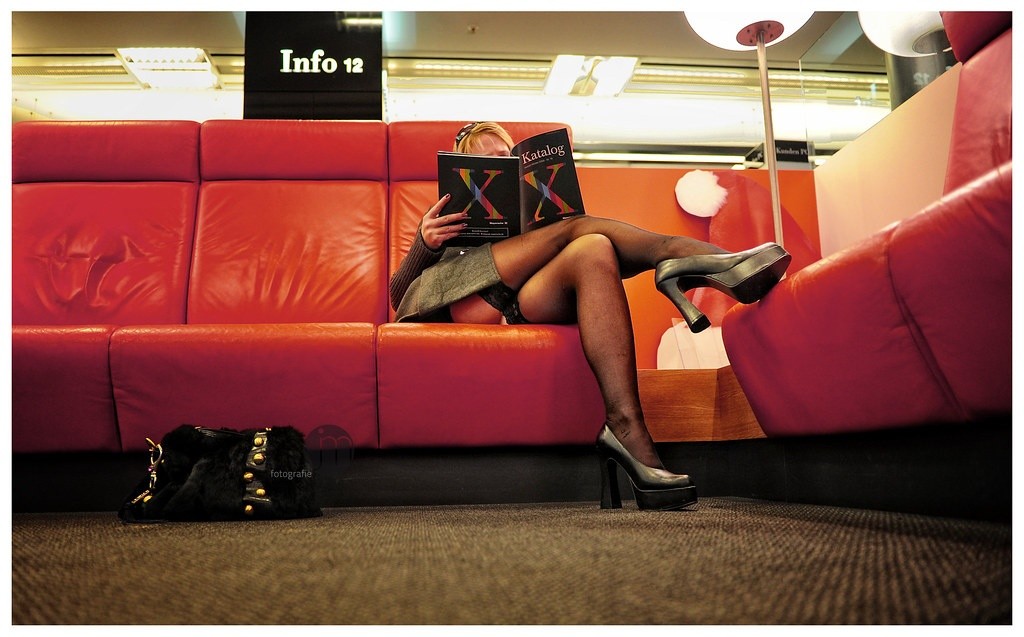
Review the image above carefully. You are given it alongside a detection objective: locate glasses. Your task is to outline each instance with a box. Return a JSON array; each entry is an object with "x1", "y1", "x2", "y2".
[{"x1": 456, "y1": 122, "x2": 485, "y2": 149}]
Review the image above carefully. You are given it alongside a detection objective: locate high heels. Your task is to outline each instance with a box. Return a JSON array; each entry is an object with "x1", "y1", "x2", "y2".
[
  {"x1": 654, "y1": 242, "x2": 792, "y2": 333},
  {"x1": 597, "y1": 423, "x2": 698, "y2": 511}
]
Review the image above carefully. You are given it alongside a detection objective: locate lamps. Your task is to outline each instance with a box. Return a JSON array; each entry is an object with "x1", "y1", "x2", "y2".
[
  {"x1": 113, "y1": 46, "x2": 226, "y2": 92},
  {"x1": 858, "y1": 11, "x2": 952, "y2": 57},
  {"x1": 541, "y1": 54, "x2": 643, "y2": 108},
  {"x1": 682, "y1": 11, "x2": 817, "y2": 282}
]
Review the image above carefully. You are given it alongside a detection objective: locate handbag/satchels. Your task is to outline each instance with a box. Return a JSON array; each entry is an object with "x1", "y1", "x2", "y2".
[{"x1": 119, "y1": 424, "x2": 321, "y2": 522}]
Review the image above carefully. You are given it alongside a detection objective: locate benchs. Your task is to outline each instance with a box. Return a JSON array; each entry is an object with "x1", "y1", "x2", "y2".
[{"x1": 12, "y1": 30, "x2": 1012, "y2": 513}]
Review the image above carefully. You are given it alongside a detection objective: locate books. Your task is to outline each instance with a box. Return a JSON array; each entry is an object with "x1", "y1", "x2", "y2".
[{"x1": 436, "y1": 128, "x2": 586, "y2": 248}]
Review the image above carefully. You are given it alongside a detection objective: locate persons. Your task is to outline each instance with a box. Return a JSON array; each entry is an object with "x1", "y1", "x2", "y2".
[{"x1": 389, "y1": 121, "x2": 792, "y2": 512}]
